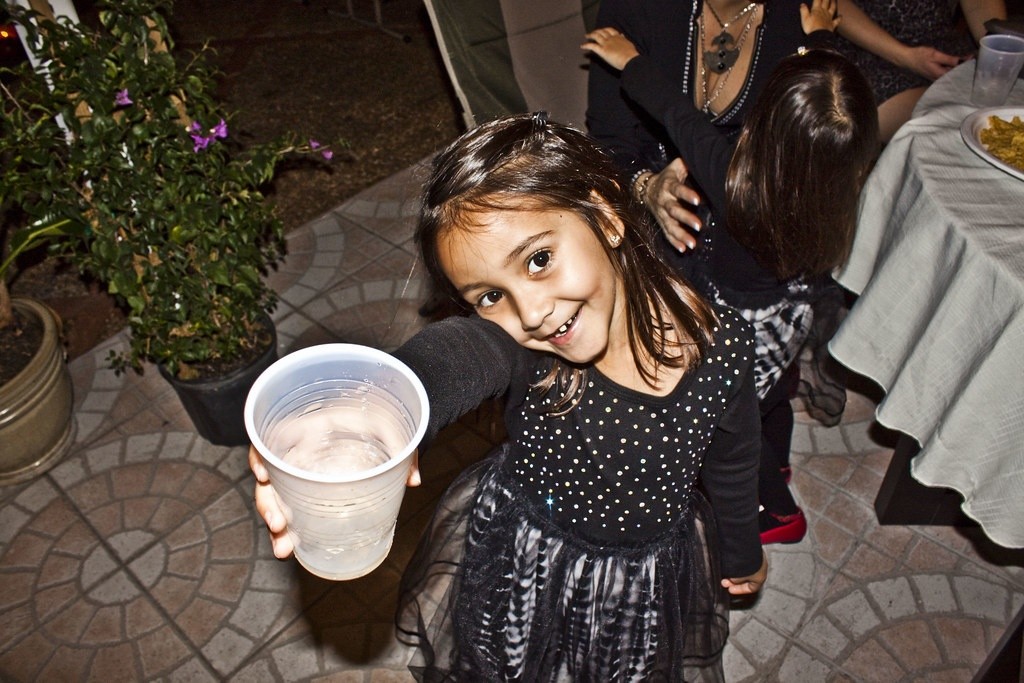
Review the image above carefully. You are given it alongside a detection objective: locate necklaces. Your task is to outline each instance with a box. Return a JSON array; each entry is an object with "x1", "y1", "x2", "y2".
[{"x1": 700, "y1": 0, "x2": 761, "y2": 117}]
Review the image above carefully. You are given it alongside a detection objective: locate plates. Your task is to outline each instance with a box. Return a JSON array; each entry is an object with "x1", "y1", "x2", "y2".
[{"x1": 960, "y1": 107, "x2": 1024, "y2": 181}]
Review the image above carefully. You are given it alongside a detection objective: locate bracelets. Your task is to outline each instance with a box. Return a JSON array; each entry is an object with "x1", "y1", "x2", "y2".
[{"x1": 631, "y1": 167, "x2": 659, "y2": 204}]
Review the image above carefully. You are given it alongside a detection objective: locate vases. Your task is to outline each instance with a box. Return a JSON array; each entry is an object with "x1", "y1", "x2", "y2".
[
  {"x1": 0, "y1": 299, "x2": 77, "y2": 487},
  {"x1": 159, "y1": 308, "x2": 278, "y2": 447}
]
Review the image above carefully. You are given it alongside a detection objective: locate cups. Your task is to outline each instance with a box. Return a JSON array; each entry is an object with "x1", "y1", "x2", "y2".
[
  {"x1": 971, "y1": 34, "x2": 1024, "y2": 108},
  {"x1": 243, "y1": 343, "x2": 431, "y2": 580}
]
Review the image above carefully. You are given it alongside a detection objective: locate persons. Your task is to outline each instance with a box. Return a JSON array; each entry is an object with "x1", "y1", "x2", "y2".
[
  {"x1": 585, "y1": 0, "x2": 847, "y2": 428},
  {"x1": 248, "y1": 111, "x2": 768, "y2": 683},
  {"x1": 580, "y1": 0, "x2": 881, "y2": 546},
  {"x1": 835, "y1": 0, "x2": 1009, "y2": 147}
]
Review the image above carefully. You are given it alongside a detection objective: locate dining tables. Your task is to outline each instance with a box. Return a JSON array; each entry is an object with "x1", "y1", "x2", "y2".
[{"x1": 874, "y1": 59, "x2": 1024, "y2": 528}]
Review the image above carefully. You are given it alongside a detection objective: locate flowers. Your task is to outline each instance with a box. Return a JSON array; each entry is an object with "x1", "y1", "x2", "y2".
[{"x1": 0, "y1": 0, "x2": 335, "y2": 377}]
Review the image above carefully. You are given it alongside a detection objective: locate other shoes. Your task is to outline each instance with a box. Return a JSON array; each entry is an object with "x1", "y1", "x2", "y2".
[
  {"x1": 756, "y1": 508, "x2": 808, "y2": 545},
  {"x1": 781, "y1": 466, "x2": 793, "y2": 483},
  {"x1": 718, "y1": 583, "x2": 761, "y2": 610}
]
